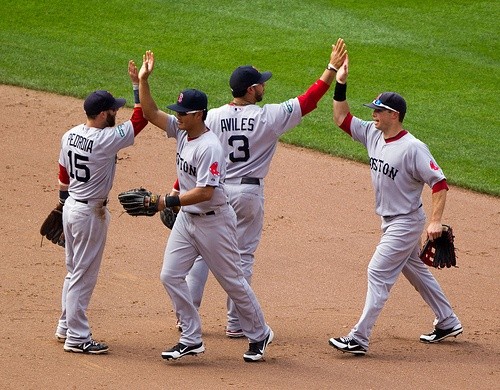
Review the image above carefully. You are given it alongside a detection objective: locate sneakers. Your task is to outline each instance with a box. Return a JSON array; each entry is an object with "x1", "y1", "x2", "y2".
[
  {"x1": 420, "y1": 323, "x2": 463, "y2": 343},
  {"x1": 56, "y1": 333, "x2": 93, "y2": 343},
  {"x1": 243, "y1": 328, "x2": 275, "y2": 363},
  {"x1": 176, "y1": 319, "x2": 182, "y2": 328},
  {"x1": 226, "y1": 328, "x2": 245, "y2": 337},
  {"x1": 161, "y1": 341, "x2": 205, "y2": 361},
  {"x1": 63, "y1": 338, "x2": 109, "y2": 354},
  {"x1": 328, "y1": 336, "x2": 367, "y2": 355}
]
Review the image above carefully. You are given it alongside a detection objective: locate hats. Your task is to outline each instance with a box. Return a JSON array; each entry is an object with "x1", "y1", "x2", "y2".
[
  {"x1": 229, "y1": 65, "x2": 272, "y2": 91},
  {"x1": 363, "y1": 91, "x2": 407, "y2": 114},
  {"x1": 83, "y1": 90, "x2": 126, "y2": 114},
  {"x1": 167, "y1": 88, "x2": 208, "y2": 112}
]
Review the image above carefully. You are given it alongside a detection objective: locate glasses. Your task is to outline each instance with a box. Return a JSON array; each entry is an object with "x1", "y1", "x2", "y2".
[
  {"x1": 111, "y1": 108, "x2": 119, "y2": 112},
  {"x1": 178, "y1": 110, "x2": 205, "y2": 116},
  {"x1": 372, "y1": 99, "x2": 400, "y2": 114}
]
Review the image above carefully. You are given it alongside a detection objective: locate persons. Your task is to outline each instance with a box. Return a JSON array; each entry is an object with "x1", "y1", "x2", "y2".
[
  {"x1": 117, "y1": 50, "x2": 273, "y2": 363},
  {"x1": 40, "y1": 60, "x2": 149, "y2": 354},
  {"x1": 176, "y1": 37, "x2": 347, "y2": 337},
  {"x1": 328, "y1": 53, "x2": 464, "y2": 356}
]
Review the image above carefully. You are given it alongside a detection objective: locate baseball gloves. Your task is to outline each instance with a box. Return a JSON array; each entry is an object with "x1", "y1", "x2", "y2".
[
  {"x1": 161, "y1": 208, "x2": 177, "y2": 230},
  {"x1": 118, "y1": 187, "x2": 161, "y2": 216},
  {"x1": 40, "y1": 206, "x2": 65, "y2": 247},
  {"x1": 418, "y1": 225, "x2": 456, "y2": 268}
]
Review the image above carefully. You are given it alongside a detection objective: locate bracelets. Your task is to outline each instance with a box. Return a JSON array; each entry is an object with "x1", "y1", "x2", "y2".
[
  {"x1": 164, "y1": 194, "x2": 181, "y2": 209},
  {"x1": 327, "y1": 63, "x2": 338, "y2": 72},
  {"x1": 59, "y1": 190, "x2": 69, "y2": 199},
  {"x1": 133, "y1": 84, "x2": 140, "y2": 103}
]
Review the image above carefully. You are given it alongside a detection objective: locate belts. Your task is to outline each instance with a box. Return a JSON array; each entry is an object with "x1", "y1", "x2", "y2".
[
  {"x1": 189, "y1": 202, "x2": 230, "y2": 216},
  {"x1": 75, "y1": 199, "x2": 107, "y2": 206},
  {"x1": 223, "y1": 177, "x2": 260, "y2": 185}
]
